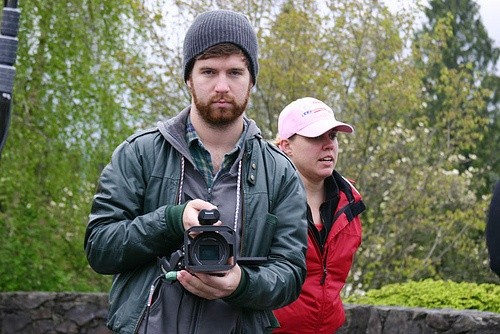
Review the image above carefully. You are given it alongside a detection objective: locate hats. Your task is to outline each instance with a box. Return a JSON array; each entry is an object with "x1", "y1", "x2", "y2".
[
  {"x1": 277, "y1": 97, "x2": 353, "y2": 143},
  {"x1": 182, "y1": 9, "x2": 259, "y2": 87}
]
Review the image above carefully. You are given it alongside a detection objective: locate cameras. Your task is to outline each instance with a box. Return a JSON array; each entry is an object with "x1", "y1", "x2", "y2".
[{"x1": 160, "y1": 209, "x2": 236, "y2": 284}]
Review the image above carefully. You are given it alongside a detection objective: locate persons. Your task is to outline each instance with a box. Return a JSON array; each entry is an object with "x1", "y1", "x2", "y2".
[
  {"x1": 82, "y1": 9, "x2": 310, "y2": 334},
  {"x1": 265, "y1": 96, "x2": 366, "y2": 334}
]
